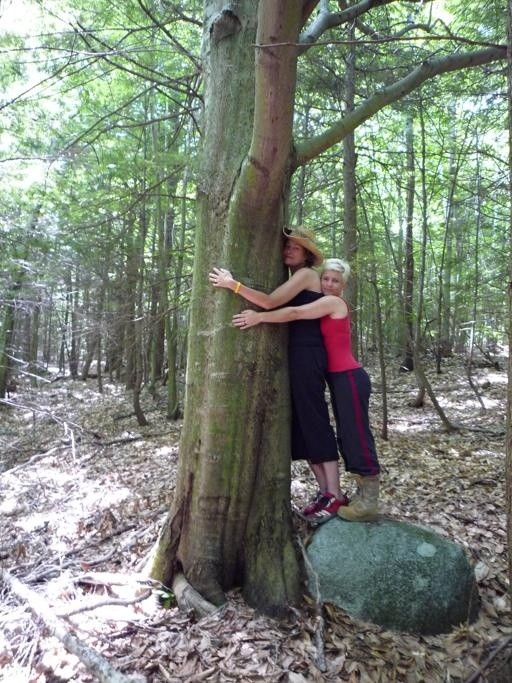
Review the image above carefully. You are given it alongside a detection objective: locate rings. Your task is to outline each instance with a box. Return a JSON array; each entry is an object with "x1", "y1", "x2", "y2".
[
  {"x1": 215, "y1": 278, "x2": 217, "y2": 282},
  {"x1": 244, "y1": 320, "x2": 247, "y2": 326}
]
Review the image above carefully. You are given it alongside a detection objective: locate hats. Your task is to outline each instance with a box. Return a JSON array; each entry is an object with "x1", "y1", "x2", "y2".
[{"x1": 282, "y1": 222, "x2": 323, "y2": 269}]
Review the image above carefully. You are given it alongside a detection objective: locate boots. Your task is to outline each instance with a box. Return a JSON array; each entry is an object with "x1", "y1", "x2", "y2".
[{"x1": 336, "y1": 473, "x2": 380, "y2": 523}]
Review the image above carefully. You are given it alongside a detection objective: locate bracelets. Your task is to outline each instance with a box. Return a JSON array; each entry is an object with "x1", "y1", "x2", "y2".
[{"x1": 235, "y1": 282, "x2": 241, "y2": 294}]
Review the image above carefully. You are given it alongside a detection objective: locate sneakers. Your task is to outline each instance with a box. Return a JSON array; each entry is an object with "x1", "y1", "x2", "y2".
[{"x1": 300, "y1": 491, "x2": 350, "y2": 524}]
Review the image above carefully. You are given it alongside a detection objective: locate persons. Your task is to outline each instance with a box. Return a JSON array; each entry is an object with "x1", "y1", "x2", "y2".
[
  {"x1": 207, "y1": 225, "x2": 351, "y2": 527},
  {"x1": 229, "y1": 257, "x2": 380, "y2": 522}
]
